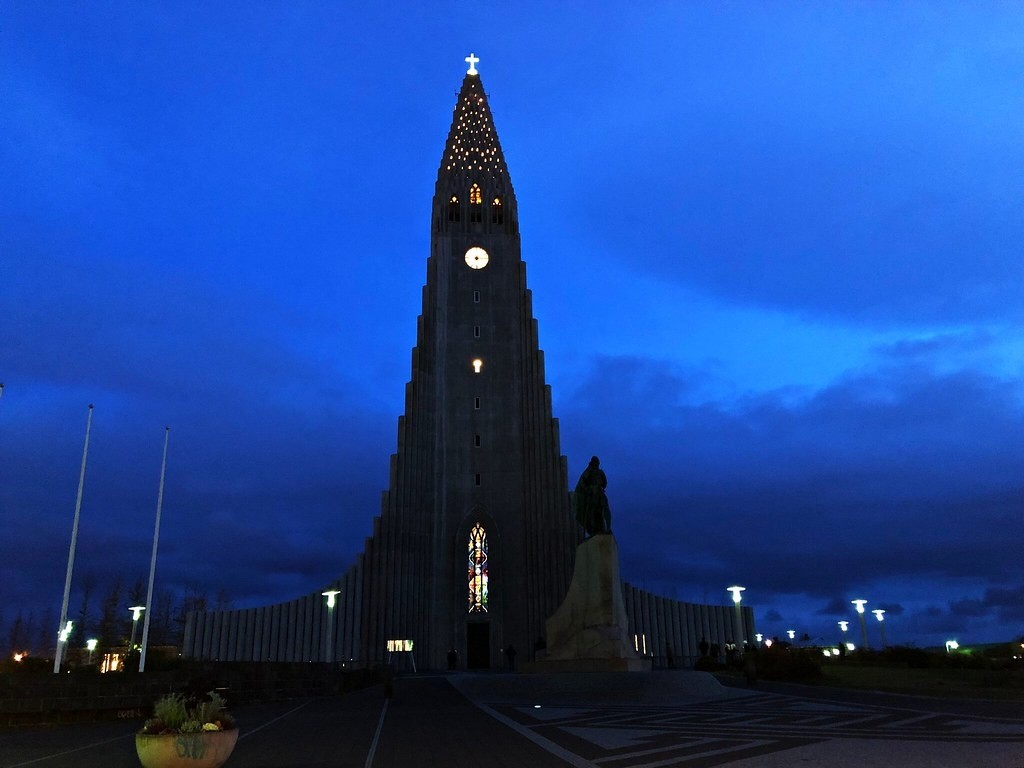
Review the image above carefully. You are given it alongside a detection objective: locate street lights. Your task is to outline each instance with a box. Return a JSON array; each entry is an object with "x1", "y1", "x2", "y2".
[
  {"x1": 727, "y1": 586, "x2": 748, "y2": 652},
  {"x1": 837, "y1": 620, "x2": 850, "y2": 655},
  {"x1": 786, "y1": 630, "x2": 795, "y2": 651},
  {"x1": 85, "y1": 636, "x2": 98, "y2": 662},
  {"x1": 128, "y1": 605, "x2": 146, "y2": 653},
  {"x1": 851, "y1": 599, "x2": 871, "y2": 647},
  {"x1": 104, "y1": 651, "x2": 111, "y2": 673},
  {"x1": 872, "y1": 608, "x2": 885, "y2": 644},
  {"x1": 320, "y1": 585, "x2": 341, "y2": 663}
]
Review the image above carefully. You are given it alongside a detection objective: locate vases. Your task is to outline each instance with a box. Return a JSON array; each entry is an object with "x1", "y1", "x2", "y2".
[{"x1": 135, "y1": 728, "x2": 239, "y2": 768}]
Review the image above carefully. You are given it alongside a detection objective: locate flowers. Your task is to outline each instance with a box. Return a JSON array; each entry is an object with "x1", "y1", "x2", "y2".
[{"x1": 140, "y1": 690, "x2": 237, "y2": 735}]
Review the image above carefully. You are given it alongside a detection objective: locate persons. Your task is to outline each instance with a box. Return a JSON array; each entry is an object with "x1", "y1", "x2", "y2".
[
  {"x1": 505, "y1": 644, "x2": 518, "y2": 672},
  {"x1": 574, "y1": 456, "x2": 612, "y2": 537},
  {"x1": 666, "y1": 642, "x2": 675, "y2": 672},
  {"x1": 535, "y1": 637, "x2": 546, "y2": 657},
  {"x1": 447, "y1": 647, "x2": 458, "y2": 671},
  {"x1": 699, "y1": 636, "x2": 846, "y2": 663}
]
